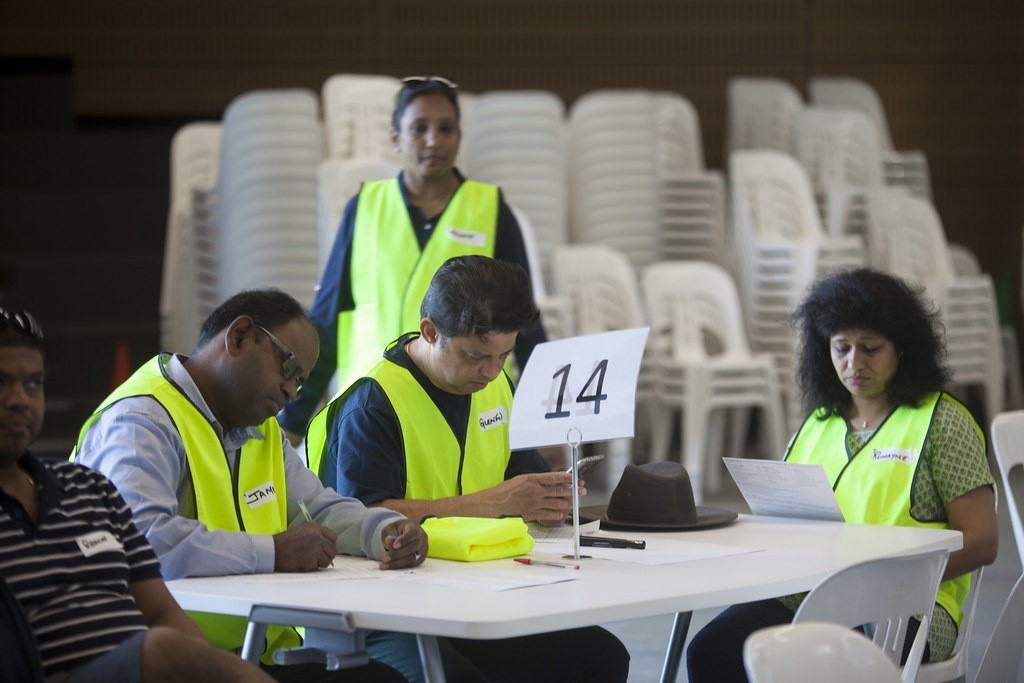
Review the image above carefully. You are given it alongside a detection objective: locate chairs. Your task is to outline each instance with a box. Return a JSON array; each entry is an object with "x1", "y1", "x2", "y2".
[
  {"x1": 160, "y1": 72, "x2": 1024, "y2": 506},
  {"x1": 990, "y1": 408, "x2": 1024, "y2": 569},
  {"x1": 743, "y1": 623, "x2": 902, "y2": 683},
  {"x1": 790, "y1": 547, "x2": 950, "y2": 683}
]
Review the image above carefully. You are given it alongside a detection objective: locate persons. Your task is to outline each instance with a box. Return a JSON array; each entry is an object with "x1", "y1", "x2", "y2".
[
  {"x1": 66, "y1": 289, "x2": 430, "y2": 683},
  {"x1": 275, "y1": 78, "x2": 553, "y2": 448},
  {"x1": 1, "y1": 303, "x2": 281, "y2": 683},
  {"x1": 686, "y1": 267, "x2": 998, "y2": 683},
  {"x1": 302, "y1": 256, "x2": 633, "y2": 683}
]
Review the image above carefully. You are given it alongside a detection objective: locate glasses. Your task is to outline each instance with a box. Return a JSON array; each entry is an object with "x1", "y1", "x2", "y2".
[
  {"x1": 253, "y1": 324, "x2": 304, "y2": 397},
  {"x1": 0, "y1": 307, "x2": 44, "y2": 339},
  {"x1": 399, "y1": 76, "x2": 459, "y2": 89}
]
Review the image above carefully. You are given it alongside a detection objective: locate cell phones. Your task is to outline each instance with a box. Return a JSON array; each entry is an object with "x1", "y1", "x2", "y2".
[{"x1": 562, "y1": 453, "x2": 607, "y2": 484}]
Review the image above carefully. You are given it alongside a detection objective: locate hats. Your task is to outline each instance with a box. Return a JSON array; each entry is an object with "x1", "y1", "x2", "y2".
[{"x1": 566, "y1": 459, "x2": 737, "y2": 529}]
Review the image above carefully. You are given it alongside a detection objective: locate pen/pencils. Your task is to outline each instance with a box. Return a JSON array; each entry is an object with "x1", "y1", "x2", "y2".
[
  {"x1": 296, "y1": 497, "x2": 336, "y2": 567},
  {"x1": 580, "y1": 535, "x2": 646, "y2": 549},
  {"x1": 513, "y1": 558, "x2": 581, "y2": 571}
]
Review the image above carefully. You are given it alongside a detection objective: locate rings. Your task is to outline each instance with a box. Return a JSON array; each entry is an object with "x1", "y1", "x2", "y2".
[{"x1": 413, "y1": 552, "x2": 421, "y2": 560}]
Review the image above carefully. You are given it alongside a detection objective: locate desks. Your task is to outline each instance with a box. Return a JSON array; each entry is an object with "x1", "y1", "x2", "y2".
[{"x1": 164, "y1": 513, "x2": 963, "y2": 683}]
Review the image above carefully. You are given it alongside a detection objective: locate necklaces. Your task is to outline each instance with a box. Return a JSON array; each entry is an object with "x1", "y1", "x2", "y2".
[
  {"x1": 853, "y1": 406, "x2": 894, "y2": 428},
  {"x1": 17, "y1": 468, "x2": 34, "y2": 486}
]
